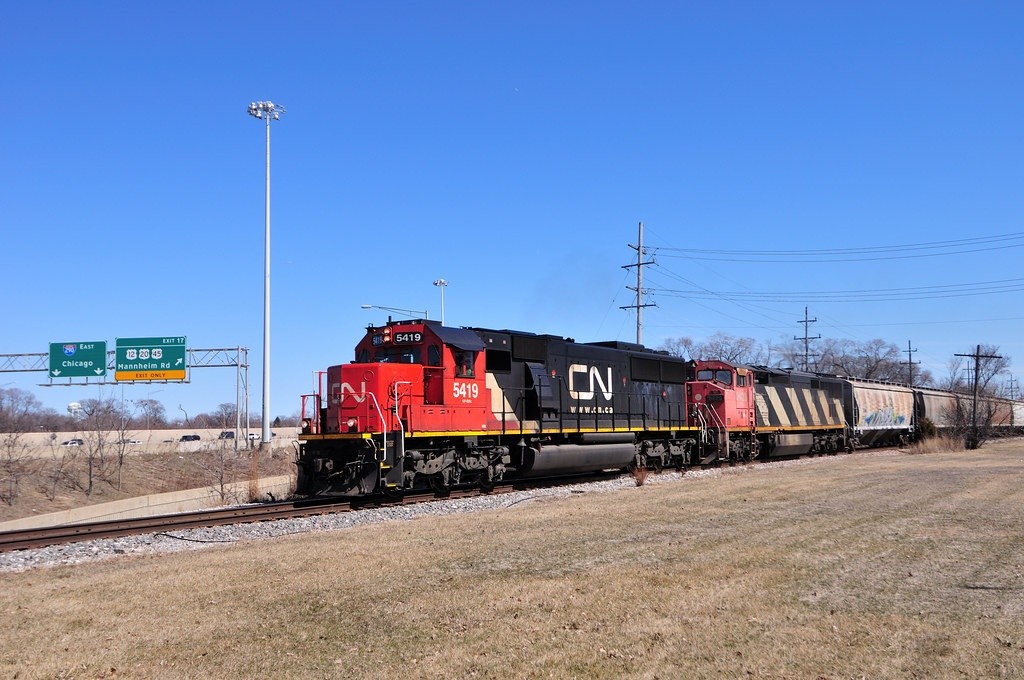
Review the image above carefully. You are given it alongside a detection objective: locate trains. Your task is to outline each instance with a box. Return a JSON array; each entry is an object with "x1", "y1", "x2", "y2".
[{"x1": 293, "y1": 322, "x2": 1024, "y2": 510}]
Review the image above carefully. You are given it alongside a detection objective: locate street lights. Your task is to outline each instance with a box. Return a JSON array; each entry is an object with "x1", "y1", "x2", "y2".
[
  {"x1": 246, "y1": 98, "x2": 288, "y2": 456},
  {"x1": 360, "y1": 304, "x2": 428, "y2": 320},
  {"x1": 432, "y1": 278, "x2": 449, "y2": 327}
]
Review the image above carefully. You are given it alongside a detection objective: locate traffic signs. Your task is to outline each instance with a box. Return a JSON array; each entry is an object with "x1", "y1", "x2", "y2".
[
  {"x1": 49, "y1": 341, "x2": 107, "y2": 377},
  {"x1": 114, "y1": 336, "x2": 186, "y2": 380}
]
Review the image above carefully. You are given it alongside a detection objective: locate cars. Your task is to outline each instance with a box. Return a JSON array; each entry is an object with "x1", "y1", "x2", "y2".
[
  {"x1": 116, "y1": 439, "x2": 142, "y2": 445},
  {"x1": 245, "y1": 433, "x2": 261, "y2": 440},
  {"x1": 61, "y1": 438, "x2": 84, "y2": 446}
]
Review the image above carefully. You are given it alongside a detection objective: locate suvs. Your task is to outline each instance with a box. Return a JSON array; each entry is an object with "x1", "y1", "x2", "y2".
[
  {"x1": 179, "y1": 434, "x2": 200, "y2": 442},
  {"x1": 218, "y1": 431, "x2": 234, "y2": 439}
]
